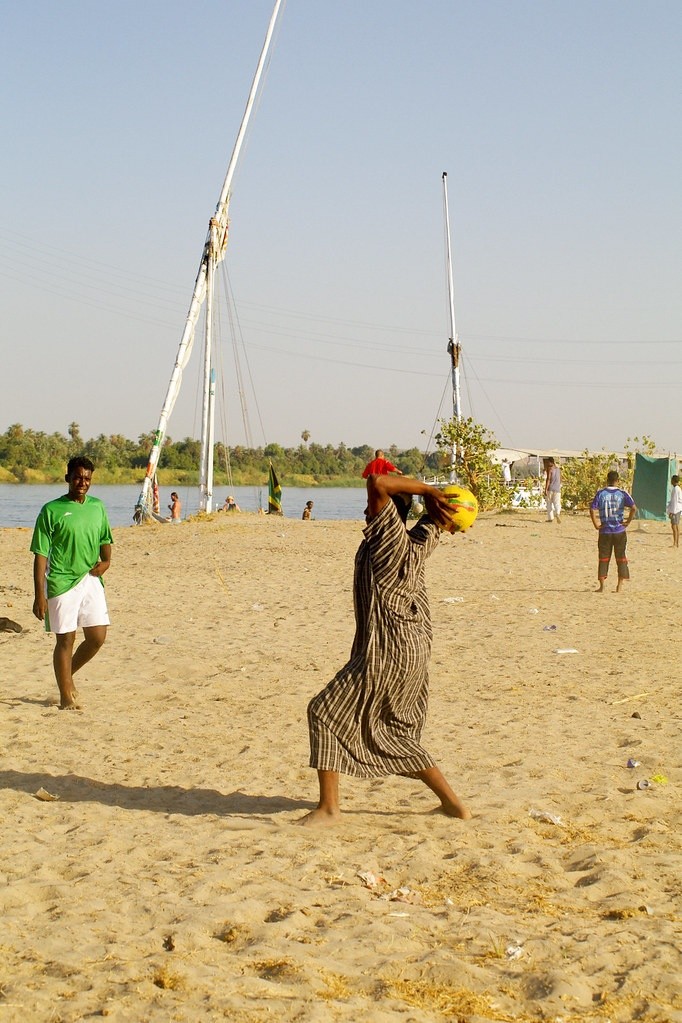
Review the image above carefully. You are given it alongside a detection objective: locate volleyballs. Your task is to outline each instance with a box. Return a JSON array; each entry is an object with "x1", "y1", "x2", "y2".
[{"x1": 434, "y1": 484, "x2": 480, "y2": 533}]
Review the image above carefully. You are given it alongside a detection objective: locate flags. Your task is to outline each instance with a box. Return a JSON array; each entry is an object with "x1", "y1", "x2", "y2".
[{"x1": 268, "y1": 464, "x2": 282, "y2": 512}]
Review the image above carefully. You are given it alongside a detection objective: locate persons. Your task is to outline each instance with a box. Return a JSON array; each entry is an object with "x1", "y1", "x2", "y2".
[
  {"x1": 167, "y1": 492, "x2": 182, "y2": 524},
  {"x1": 544, "y1": 456, "x2": 563, "y2": 525},
  {"x1": 361, "y1": 450, "x2": 403, "y2": 478},
  {"x1": 218, "y1": 495, "x2": 241, "y2": 514},
  {"x1": 30, "y1": 456, "x2": 114, "y2": 710},
  {"x1": 290, "y1": 475, "x2": 473, "y2": 827},
  {"x1": 302, "y1": 501, "x2": 313, "y2": 520},
  {"x1": 667, "y1": 474, "x2": 682, "y2": 550},
  {"x1": 589, "y1": 470, "x2": 636, "y2": 593}
]
